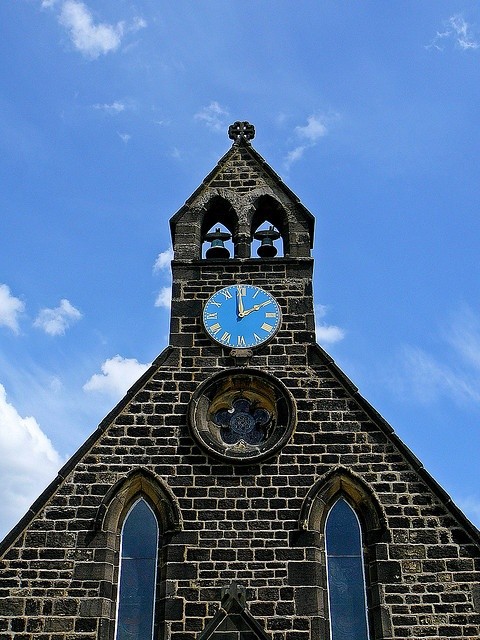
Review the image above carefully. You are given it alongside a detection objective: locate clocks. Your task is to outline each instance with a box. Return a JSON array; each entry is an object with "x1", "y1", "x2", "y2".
[{"x1": 199, "y1": 281, "x2": 285, "y2": 361}]
